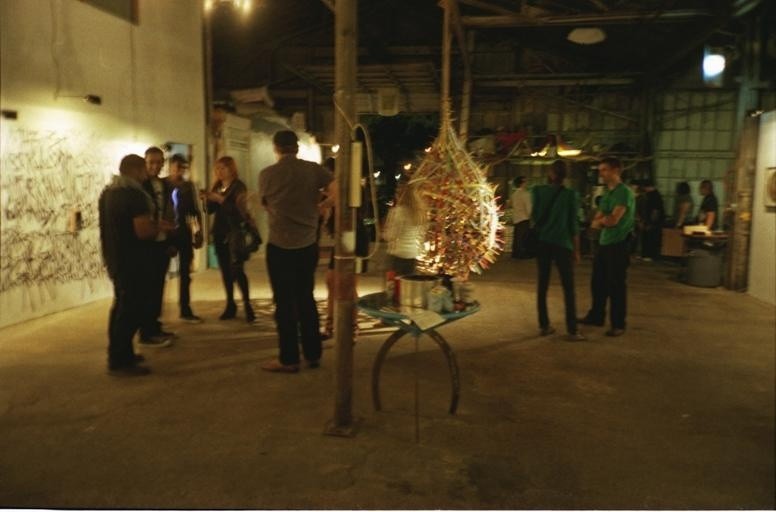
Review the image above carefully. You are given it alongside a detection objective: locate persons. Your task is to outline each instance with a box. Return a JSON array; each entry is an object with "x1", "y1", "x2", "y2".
[
  {"x1": 314, "y1": 156, "x2": 378, "y2": 273},
  {"x1": 530, "y1": 160, "x2": 588, "y2": 343},
  {"x1": 256, "y1": 130, "x2": 333, "y2": 373},
  {"x1": 511, "y1": 176, "x2": 719, "y2": 263},
  {"x1": 136, "y1": 147, "x2": 179, "y2": 349},
  {"x1": 576, "y1": 157, "x2": 636, "y2": 337},
  {"x1": 98, "y1": 155, "x2": 171, "y2": 376},
  {"x1": 154, "y1": 153, "x2": 203, "y2": 325},
  {"x1": 200, "y1": 156, "x2": 256, "y2": 323}
]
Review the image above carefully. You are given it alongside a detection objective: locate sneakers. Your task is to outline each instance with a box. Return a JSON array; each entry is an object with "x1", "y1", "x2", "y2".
[
  {"x1": 300, "y1": 358, "x2": 321, "y2": 369},
  {"x1": 137, "y1": 313, "x2": 256, "y2": 347},
  {"x1": 107, "y1": 354, "x2": 150, "y2": 377},
  {"x1": 261, "y1": 358, "x2": 300, "y2": 373},
  {"x1": 540, "y1": 316, "x2": 625, "y2": 342}
]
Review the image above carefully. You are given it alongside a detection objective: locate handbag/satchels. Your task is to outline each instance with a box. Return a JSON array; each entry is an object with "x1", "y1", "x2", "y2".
[
  {"x1": 518, "y1": 229, "x2": 538, "y2": 258},
  {"x1": 224, "y1": 223, "x2": 263, "y2": 265}
]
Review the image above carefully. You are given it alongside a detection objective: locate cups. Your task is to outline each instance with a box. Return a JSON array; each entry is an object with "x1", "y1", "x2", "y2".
[{"x1": 384, "y1": 273, "x2": 476, "y2": 313}]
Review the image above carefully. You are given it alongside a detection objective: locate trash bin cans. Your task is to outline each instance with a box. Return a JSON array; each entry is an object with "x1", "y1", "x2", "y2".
[{"x1": 681, "y1": 231, "x2": 728, "y2": 288}]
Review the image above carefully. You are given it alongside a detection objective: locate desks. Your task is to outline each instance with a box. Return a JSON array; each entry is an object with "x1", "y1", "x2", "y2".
[{"x1": 356, "y1": 289, "x2": 480, "y2": 417}]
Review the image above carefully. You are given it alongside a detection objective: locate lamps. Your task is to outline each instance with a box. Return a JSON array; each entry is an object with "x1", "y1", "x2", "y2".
[{"x1": 53, "y1": 90, "x2": 102, "y2": 107}]
